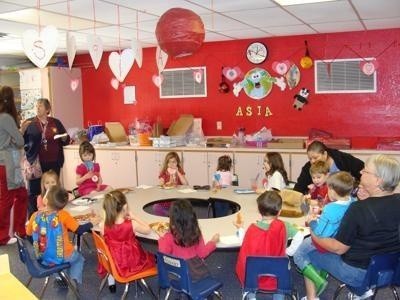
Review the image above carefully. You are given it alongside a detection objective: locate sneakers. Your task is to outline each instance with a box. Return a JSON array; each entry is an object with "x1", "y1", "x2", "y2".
[
  {"x1": 301, "y1": 296, "x2": 320, "y2": 300},
  {"x1": 348, "y1": 288, "x2": 374, "y2": 299},
  {"x1": 6, "y1": 238, "x2": 18, "y2": 244}
]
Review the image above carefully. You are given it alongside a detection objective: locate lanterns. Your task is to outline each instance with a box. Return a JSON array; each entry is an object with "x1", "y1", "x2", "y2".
[{"x1": 155, "y1": 8, "x2": 206, "y2": 58}]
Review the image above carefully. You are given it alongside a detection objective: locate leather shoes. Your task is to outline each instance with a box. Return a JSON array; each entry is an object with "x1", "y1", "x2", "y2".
[
  {"x1": 55, "y1": 279, "x2": 66, "y2": 290},
  {"x1": 67, "y1": 279, "x2": 77, "y2": 300},
  {"x1": 109, "y1": 285, "x2": 116, "y2": 292}
]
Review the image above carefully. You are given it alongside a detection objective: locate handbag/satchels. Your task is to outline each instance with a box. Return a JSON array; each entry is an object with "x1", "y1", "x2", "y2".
[{"x1": 24, "y1": 155, "x2": 42, "y2": 180}]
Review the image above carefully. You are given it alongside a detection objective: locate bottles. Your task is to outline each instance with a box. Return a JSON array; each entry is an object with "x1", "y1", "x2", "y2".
[
  {"x1": 309, "y1": 199, "x2": 319, "y2": 221},
  {"x1": 257, "y1": 138, "x2": 262, "y2": 148},
  {"x1": 240, "y1": 128, "x2": 246, "y2": 144}
]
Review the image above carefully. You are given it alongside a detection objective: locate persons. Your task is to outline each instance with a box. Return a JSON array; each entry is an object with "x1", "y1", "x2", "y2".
[
  {"x1": 157, "y1": 201, "x2": 220, "y2": 285},
  {"x1": 293, "y1": 172, "x2": 356, "y2": 297},
  {"x1": 293, "y1": 141, "x2": 365, "y2": 195},
  {"x1": 25, "y1": 185, "x2": 101, "y2": 294},
  {"x1": 37, "y1": 170, "x2": 74, "y2": 210},
  {"x1": 253, "y1": 153, "x2": 289, "y2": 192},
  {"x1": 300, "y1": 155, "x2": 400, "y2": 300},
  {"x1": 235, "y1": 191, "x2": 287, "y2": 300},
  {"x1": 77, "y1": 141, "x2": 113, "y2": 195},
  {"x1": 158, "y1": 152, "x2": 188, "y2": 189},
  {"x1": 23, "y1": 99, "x2": 72, "y2": 220},
  {"x1": 97, "y1": 190, "x2": 156, "y2": 292},
  {"x1": 0, "y1": 86, "x2": 30, "y2": 244},
  {"x1": 305, "y1": 162, "x2": 331, "y2": 209},
  {"x1": 212, "y1": 156, "x2": 237, "y2": 188}
]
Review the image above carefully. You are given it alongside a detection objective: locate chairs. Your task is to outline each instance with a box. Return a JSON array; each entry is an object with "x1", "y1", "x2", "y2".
[
  {"x1": 12, "y1": 230, "x2": 82, "y2": 300},
  {"x1": 242, "y1": 255, "x2": 296, "y2": 300},
  {"x1": 91, "y1": 228, "x2": 159, "y2": 300},
  {"x1": 333, "y1": 252, "x2": 400, "y2": 300},
  {"x1": 157, "y1": 250, "x2": 225, "y2": 300}
]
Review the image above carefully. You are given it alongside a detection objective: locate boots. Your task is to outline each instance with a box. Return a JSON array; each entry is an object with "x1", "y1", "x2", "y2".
[
  {"x1": 321, "y1": 270, "x2": 328, "y2": 278},
  {"x1": 302, "y1": 263, "x2": 328, "y2": 297}
]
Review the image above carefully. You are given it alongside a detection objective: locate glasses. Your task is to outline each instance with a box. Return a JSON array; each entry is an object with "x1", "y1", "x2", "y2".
[{"x1": 360, "y1": 170, "x2": 374, "y2": 175}]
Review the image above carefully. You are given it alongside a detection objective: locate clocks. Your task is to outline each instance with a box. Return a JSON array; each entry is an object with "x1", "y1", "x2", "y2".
[{"x1": 244, "y1": 41, "x2": 268, "y2": 63}]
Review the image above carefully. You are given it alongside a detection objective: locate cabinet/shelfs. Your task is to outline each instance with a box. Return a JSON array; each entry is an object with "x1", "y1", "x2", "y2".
[
  {"x1": 206, "y1": 138, "x2": 292, "y2": 191},
  {"x1": 292, "y1": 139, "x2": 400, "y2": 186},
  {"x1": 17, "y1": 69, "x2": 83, "y2": 129},
  {"x1": 137, "y1": 139, "x2": 206, "y2": 191},
  {"x1": 62, "y1": 136, "x2": 136, "y2": 194}
]
